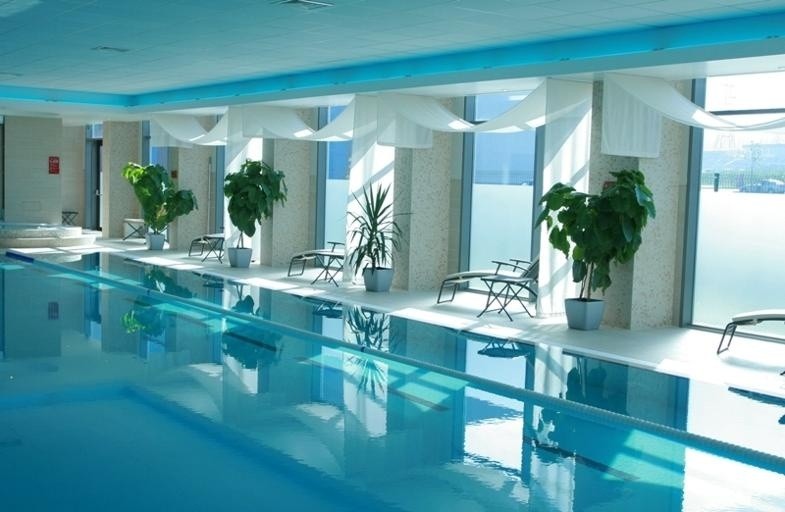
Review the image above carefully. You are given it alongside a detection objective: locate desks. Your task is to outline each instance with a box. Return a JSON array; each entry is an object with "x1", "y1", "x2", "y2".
[
  {"x1": 477, "y1": 274, "x2": 535, "y2": 322},
  {"x1": 63, "y1": 209, "x2": 80, "y2": 226},
  {"x1": 122, "y1": 217, "x2": 146, "y2": 240},
  {"x1": 310, "y1": 251, "x2": 344, "y2": 287}
]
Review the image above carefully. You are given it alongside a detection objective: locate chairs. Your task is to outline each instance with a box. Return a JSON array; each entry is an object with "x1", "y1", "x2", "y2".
[
  {"x1": 188, "y1": 226, "x2": 226, "y2": 264},
  {"x1": 192, "y1": 271, "x2": 224, "y2": 292},
  {"x1": 288, "y1": 241, "x2": 345, "y2": 287},
  {"x1": 440, "y1": 326, "x2": 531, "y2": 359},
  {"x1": 437, "y1": 253, "x2": 539, "y2": 307},
  {"x1": 727, "y1": 387, "x2": 785, "y2": 426},
  {"x1": 292, "y1": 295, "x2": 344, "y2": 319},
  {"x1": 716, "y1": 309, "x2": 784, "y2": 355}
]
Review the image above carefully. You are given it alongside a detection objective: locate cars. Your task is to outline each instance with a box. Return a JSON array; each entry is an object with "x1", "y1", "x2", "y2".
[
  {"x1": 519, "y1": 178, "x2": 533, "y2": 187},
  {"x1": 739, "y1": 178, "x2": 785, "y2": 194}
]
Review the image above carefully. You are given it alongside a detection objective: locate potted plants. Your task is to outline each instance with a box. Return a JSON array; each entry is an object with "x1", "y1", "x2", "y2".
[
  {"x1": 223, "y1": 157, "x2": 287, "y2": 267},
  {"x1": 344, "y1": 180, "x2": 416, "y2": 292},
  {"x1": 120, "y1": 159, "x2": 199, "y2": 251},
  {"x1": 341, "y1": 304, "x2": 390, "y2": 401},
  {"x1": 522, "y1": 347, "x2": 634, "y2": 478},
  {"x1": 533, "y1": 168, "x2": 656, "y2": 332},
  {"x1": 120, "y1": 263, "x2": 195, "y2": 337},
  {"x1": 215, "y1": 279, "x2": 283, "y2": 370}
]
